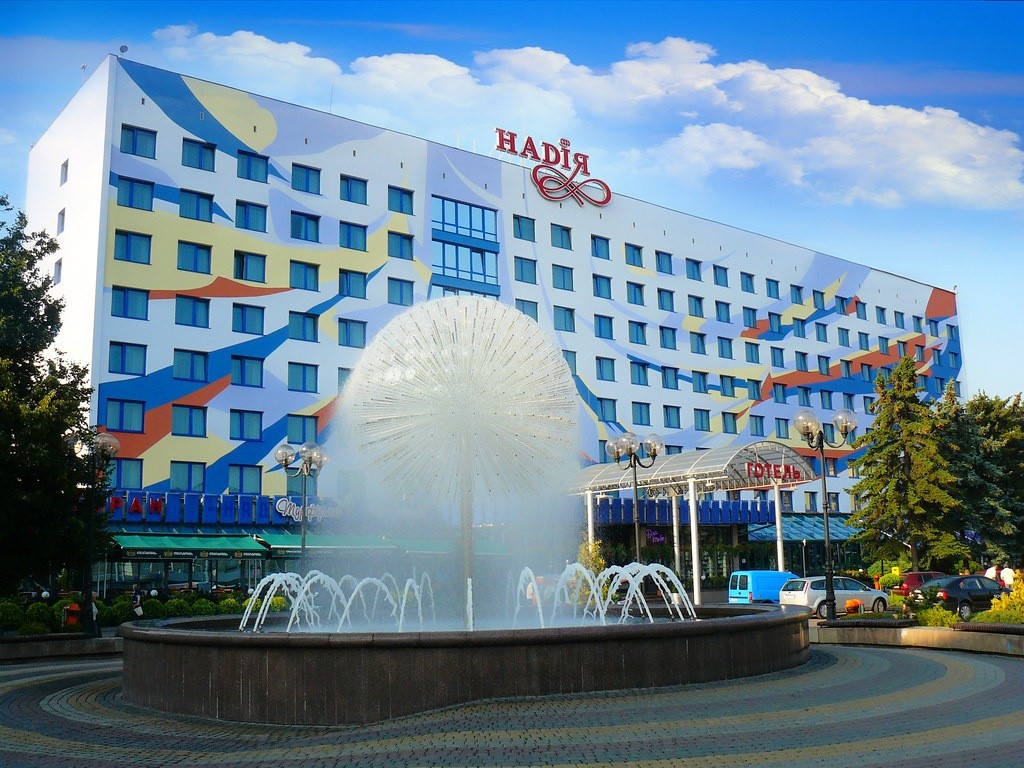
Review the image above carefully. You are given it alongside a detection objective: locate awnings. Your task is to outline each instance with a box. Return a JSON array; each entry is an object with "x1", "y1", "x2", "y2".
[
  {"x1": 111, "y1": 535, "x2": 269, "y2": 560},
  {"x1": 385, "y1": 537, "x2": 512, "y2": 557},
  {"x1": 256, "y1": 534, "x2": 398, "y2": 561}
]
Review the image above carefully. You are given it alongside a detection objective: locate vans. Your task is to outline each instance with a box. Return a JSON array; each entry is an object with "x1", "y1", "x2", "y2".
[
  {"x1": 779, "y1": 576, "x2": 889, "y2": 619},
  {"x1": 728, "y1": 570, "x2": 802, "y2": 608}
]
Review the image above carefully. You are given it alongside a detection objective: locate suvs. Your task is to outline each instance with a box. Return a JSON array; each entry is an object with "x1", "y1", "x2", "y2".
[{"x1": 892, "y1": 571, "x2": 946, "y2": 599}]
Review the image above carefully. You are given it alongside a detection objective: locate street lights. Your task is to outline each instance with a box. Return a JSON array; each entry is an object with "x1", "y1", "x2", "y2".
[
  {"x1": 605, "y1": 431, "x2": 665, "y2": 563},
  {"x1": 794, "y1": 407, "x2": 857, "y2": 620},
  {"x1": 61, "y1": 433, "x2": 120, "y2": 637},
  {"x1": 276, "y1": 442, "x2": 328, "y2": 574}
]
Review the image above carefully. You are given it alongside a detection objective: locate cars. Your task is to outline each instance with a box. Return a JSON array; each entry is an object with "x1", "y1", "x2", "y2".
[{"x1": 908, "y1": 575, "x2": 1014, "y2": 620}]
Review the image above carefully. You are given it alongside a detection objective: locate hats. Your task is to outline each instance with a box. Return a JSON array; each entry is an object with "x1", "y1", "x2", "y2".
[{"x1": 859, "y1": 568, "x2": 863, "y2": 573}]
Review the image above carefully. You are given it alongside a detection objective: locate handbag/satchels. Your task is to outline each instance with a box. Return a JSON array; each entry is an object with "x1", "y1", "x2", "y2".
[{"x1": 132, "y1": 603, "x2": 144, "y2": 616}]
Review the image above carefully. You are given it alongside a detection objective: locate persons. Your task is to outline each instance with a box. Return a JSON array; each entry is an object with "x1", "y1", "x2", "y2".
[
  {"x1": 1000, "y1": 563, "x2": 1016, "y2": 589},
  {"x1": 132, "y1": 584, "x2": 142, "y2": 620},
  {"x1": 91, "y1": 591, "x2": 102, "y2": 638},
  {"x1": 984, "y1": 564, "x2": 998, "y2": 581}
]
